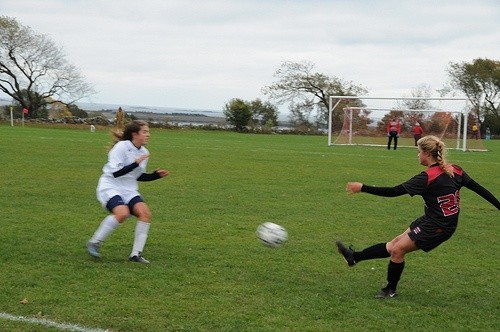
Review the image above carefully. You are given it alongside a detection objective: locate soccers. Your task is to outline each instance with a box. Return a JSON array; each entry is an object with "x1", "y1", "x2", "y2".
[{"x1": 257, "y1": 222, "x2": 288, "y2": 247}]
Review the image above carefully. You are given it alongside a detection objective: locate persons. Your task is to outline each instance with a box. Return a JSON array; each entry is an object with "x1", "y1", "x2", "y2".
[
  {"x1": 88, "y1": 120, "x2": 167, "y2": 263},
  {"x1": 472, "y1": 123, "x2": 479, "y2": 139},
  {"x1": 486, "y1": 128, "x2": 491, "y2": 139},
  {"x1": 388, "y1": 118, "x2": 400, "y2": 150},
  {"x1": 336, "y1": 136, "x2": 500, "y2": 298},
  {"x1": 413, "y1": 123, "x2": 423, "y2": 146},
  {"x1": 116, "y1": 107, "x2": 124, "y2": 129}
]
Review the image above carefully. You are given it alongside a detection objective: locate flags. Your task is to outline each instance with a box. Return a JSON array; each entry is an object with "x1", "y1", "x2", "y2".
[{"x1": 24, "y1": 109, "x2": 28, "y2": 113}]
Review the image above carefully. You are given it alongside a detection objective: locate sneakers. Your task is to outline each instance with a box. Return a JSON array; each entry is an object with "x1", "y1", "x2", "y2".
[
  {"x1": 336, "y1": 241, "x2": 357, "y2": 267},
  {"x1": 88, "y1": 242, "x2": 100, "y2": 257},
  {"x1": 130, "y1": 251, "x2": 150, "y2": 263},
  {"x1": 374, "y1": 286, "x2": 398, "y2": 300}
]
